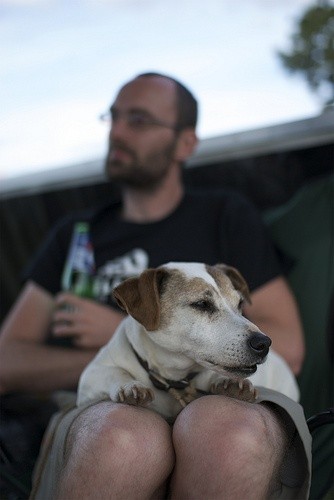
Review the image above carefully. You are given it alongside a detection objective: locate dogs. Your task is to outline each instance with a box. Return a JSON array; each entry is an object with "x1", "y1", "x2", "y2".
[{"x1": 76, "y1": 261, "x2": 272, "y2": 423}]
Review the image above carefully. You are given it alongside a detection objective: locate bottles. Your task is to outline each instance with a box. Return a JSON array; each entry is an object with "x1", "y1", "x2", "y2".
[{"x1": 62, "y1": 223, "x2": 95, "y2": 326}]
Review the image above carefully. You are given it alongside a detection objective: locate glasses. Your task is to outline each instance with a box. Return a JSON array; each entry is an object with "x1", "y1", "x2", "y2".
[{"x1": 100, "y1": 109, "x2": 180, "y2": 133}]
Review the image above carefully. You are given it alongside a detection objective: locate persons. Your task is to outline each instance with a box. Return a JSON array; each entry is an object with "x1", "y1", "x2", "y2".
[{"x1": 0, "y1": 73, "x2": 311, "y2": 500}]
